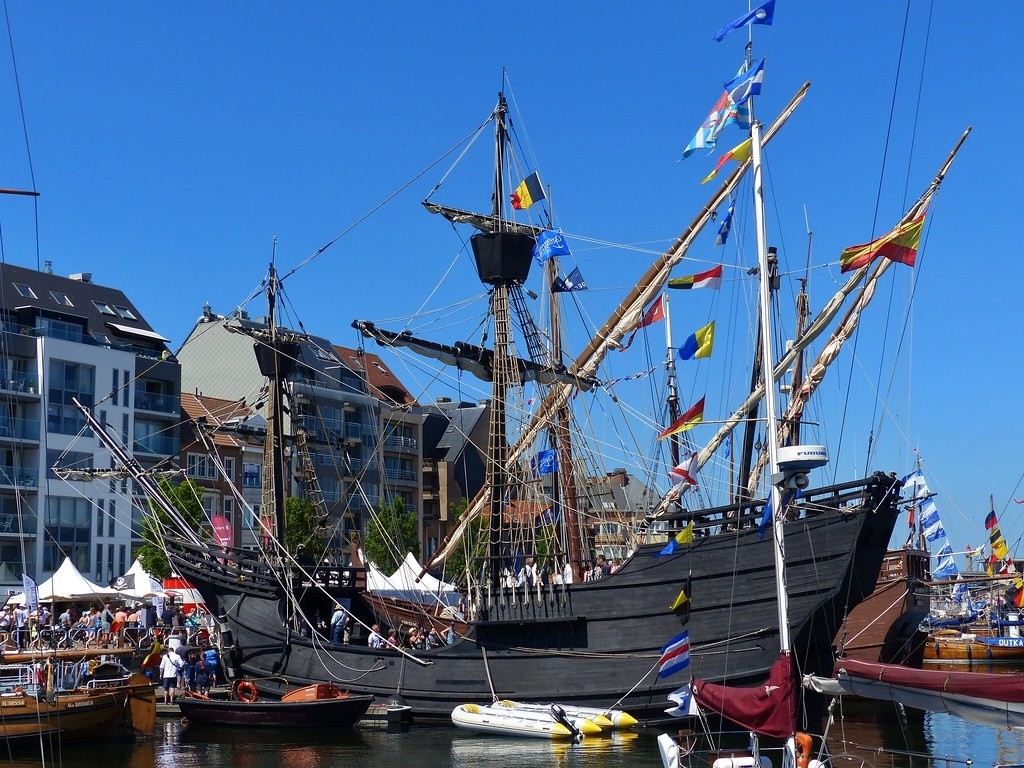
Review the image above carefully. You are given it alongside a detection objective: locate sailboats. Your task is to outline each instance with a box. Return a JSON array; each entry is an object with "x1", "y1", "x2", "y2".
[
  {"x1": 655, "y1": 119, "x2": 1024, "y2": 768},
  {"x1": 50, "y1": 1, "x2": 971, "y2": 718}
]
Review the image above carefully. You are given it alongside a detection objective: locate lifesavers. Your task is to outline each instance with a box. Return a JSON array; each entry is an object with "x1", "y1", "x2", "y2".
[
  {"x1": 237, "y1": 681, "x2": 257, "y2": 703},
  {"x1": 37, "y1": 664, "x2": 46, "y2": 687},
  {"x1": 794, "y1": 732, "x2": 813, "y2": 767}
]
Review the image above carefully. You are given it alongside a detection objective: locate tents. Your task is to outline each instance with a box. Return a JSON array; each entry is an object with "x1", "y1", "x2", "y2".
[
  {"x1": 390, "y1": 552, "x2": 457, "y2": 593},
  {"x1": 7, "y1": 556, "x2": 120, "y2": 626},
  {"x1": 337, "y1": 548, "x2": 396, "y2": 590},
  {"x1": 106, "y1": 551, "x2": 163, "y2": 608}
]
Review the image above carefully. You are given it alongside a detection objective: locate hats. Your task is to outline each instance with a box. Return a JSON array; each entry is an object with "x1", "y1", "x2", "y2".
[
  {"x1": 409, "y1": 628, "x2": 416, "y2": 634},
  {"x1": 334, "y1": 605, "x2": 343, "y2": 610},
  {"x1": 449, "y1": 621, "x2": 455, "y2": 625}
]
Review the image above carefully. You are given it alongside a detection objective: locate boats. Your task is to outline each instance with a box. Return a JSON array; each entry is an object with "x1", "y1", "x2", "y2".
[
  {"x1": 802, "y1": 573, "x2": 1024, "y2": 731},
  {"x1": 358, "y1": 590, "x2": 478, "y2": 643},
  {"x1": 0, "y1": 661, "x2": 161, "y2": 740},
  {"x1": 452, "y1": 700, "x2": 639, "y2": 739},
  {"x1": 174, "y1": 684, "x2": 375, "y2": 729},
  {"x1": 924, "y1": 614, "x2": 1024, "y2": 660}
]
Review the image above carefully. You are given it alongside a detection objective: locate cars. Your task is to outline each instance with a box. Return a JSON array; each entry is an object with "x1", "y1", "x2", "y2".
[{"x1": 969, "y1": 601, "x2": 988, "y2": 617}]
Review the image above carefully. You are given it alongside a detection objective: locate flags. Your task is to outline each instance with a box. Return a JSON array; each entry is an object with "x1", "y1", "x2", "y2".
[
  {"x1": 532, "y1": 227, "x2": 572, "y2": 268},
  {"x1": 901, "y1": 469, "x2": 1024, "y2": 617},
  {"x1": 664, "y1": 683, "x2": 700, "y2": 716},
  {"x1": 620, "y1": 293, "x2": 666, "y2": 352},
  {"x1": 677, "y1": 0, "x2": 775, "y2": 245},
  {"x1": 552, "y1": 267, "x2": 588, "y2": 295},
  {"x1": 840, "y1": 213, "x2": 926, "y2": 273},
  {"x1": 659, "y1": 630, "x2": 691, "y2": 679},
  {"x1": 677, "y1": 320, "x2": 715, "y2": 360},
  {"x1": 509, "y1": 172, "x2": 547, "y2": 209},
  {"x1": 669, "y1": 452, "x2": 698, "y2": 498},
  {"x1": 668, "y1": 265, "x2": 723, "y2": 290},
  {"x1": 531, "y1": 449, "x2": 560, "y2": 479},
  {"x1": 656, "y1": 395, "x2": 706, "y2": 441},
  {"x1": 758, "y1": 483, "x2": 801, "y2": 541},
  {"x1": 725, "y1": 435, "x2": 731, "y2": 458},
  {"x1": 668, "y1": 572, "x2": 691, "y2": 626},
  {"x1": 652, "y1": 520, "x2": 693, "y2": 558}
]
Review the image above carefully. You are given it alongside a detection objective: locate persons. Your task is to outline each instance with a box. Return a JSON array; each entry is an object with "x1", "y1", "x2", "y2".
[
  {"x1": 298, "y1": 592, "x2": 467, "y2": 650},
  {"x1": 499, "y1": 555, "x2": 622, "y2": 587},
  {"x1": 0, "y1": 604, "x2": 217, "y2": 705}
]
